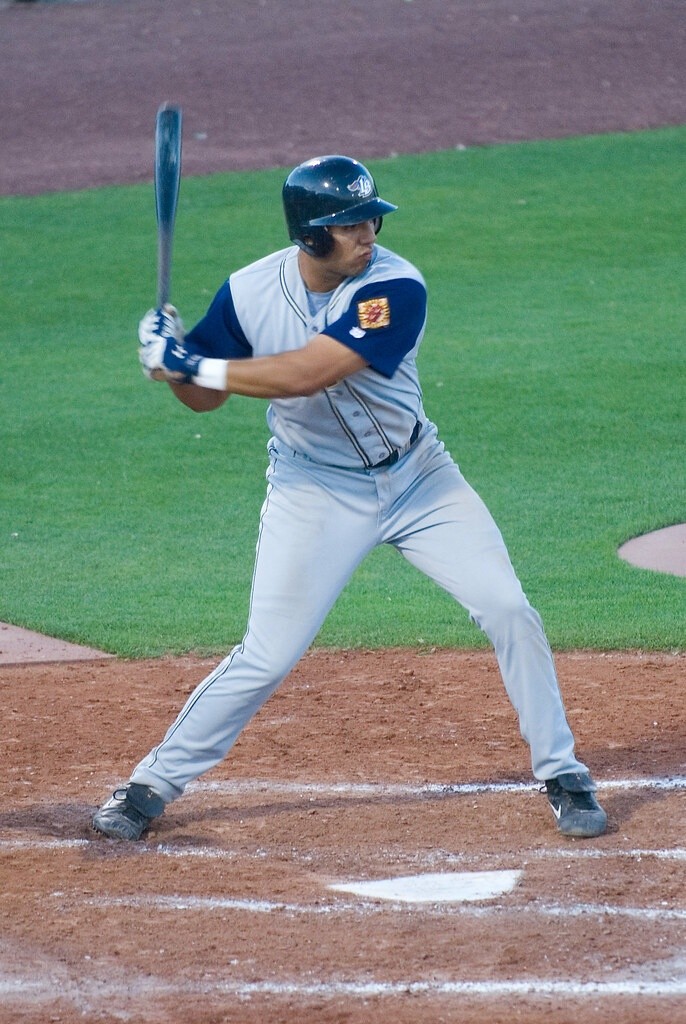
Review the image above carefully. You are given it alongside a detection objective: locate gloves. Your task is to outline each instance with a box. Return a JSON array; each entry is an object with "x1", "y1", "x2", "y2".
[
  {"x1": 138, "y1": 304, "x2": 185, "y2": 346},
  {"x1": 138, "y1": 336, "x2": 227, "y2": 392}
]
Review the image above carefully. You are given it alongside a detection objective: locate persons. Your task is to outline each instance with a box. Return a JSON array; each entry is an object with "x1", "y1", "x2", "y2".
[{"x1": 88, "y1": 156, "x2": 608, "y2": 842}]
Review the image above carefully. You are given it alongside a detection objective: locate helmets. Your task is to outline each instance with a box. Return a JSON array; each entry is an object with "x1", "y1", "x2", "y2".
[{"x1": 282, "y1": 156, "x2": 399, "y2": 257}]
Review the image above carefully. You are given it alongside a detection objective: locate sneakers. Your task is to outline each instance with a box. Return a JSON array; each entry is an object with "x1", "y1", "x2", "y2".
[
  {"x1": 93, "y1": 783, "x2": 165, "y2": 840},
  {"x1": 539, "y1": 772, "x2": 606, "y2": 836}
]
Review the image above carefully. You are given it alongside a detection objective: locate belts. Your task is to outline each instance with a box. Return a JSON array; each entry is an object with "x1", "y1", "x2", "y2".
[{"x1": 370, "y1": 420, "x2": 423, "y2": 469}]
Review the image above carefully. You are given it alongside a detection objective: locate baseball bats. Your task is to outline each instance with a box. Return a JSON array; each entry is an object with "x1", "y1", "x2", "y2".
[{"x1": 148, "y1": 100, "x2": 182, "y2": 383}]
